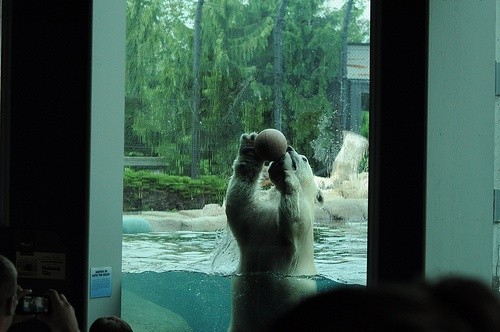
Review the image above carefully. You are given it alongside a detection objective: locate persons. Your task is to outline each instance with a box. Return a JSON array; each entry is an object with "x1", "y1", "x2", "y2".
[{"x1": 1, "y1": 237, "x2": 500, "y2": 332}]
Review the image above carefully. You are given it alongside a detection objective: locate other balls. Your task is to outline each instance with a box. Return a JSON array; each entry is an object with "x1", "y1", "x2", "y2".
[{"x1": 253, "y1": 128, "x2": 288, "y2": 162}]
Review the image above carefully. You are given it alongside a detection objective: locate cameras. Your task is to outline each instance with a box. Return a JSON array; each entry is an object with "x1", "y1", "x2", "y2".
[{"x1": 21, "y1": 294, "x2": 50, "y2": 315}]
